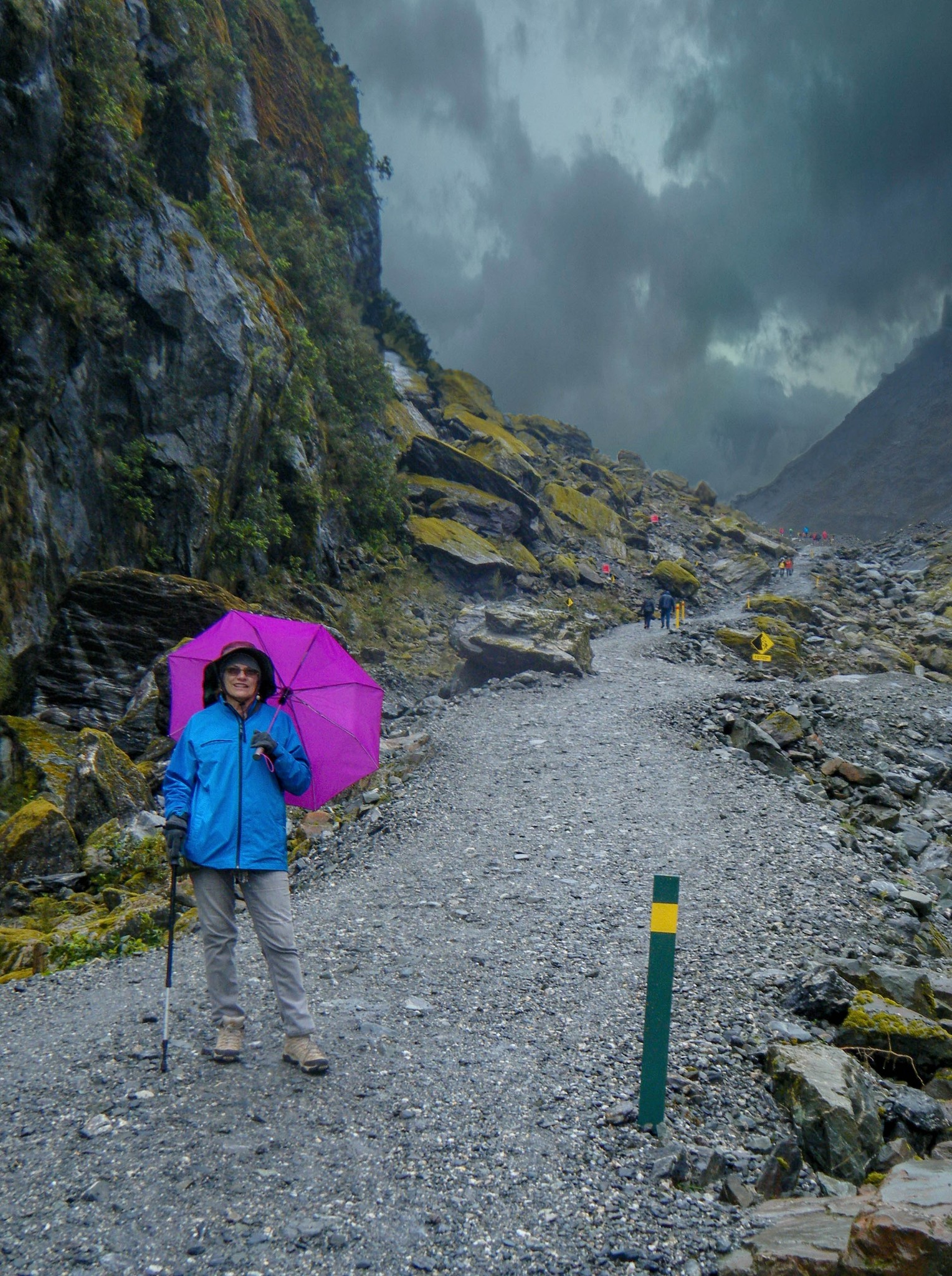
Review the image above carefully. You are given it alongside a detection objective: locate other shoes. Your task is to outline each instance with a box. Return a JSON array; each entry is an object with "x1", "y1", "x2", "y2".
[
  {"x1": 661, "y1": 625, "x2": 664, "y2": 628},
  {"x1": 644, "y1": 624, "x2": 646, "y2": 629}
]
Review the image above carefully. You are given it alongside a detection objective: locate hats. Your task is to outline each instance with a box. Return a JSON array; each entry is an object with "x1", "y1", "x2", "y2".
[
  {"x1": 203, "y1": 641, "x2": 276, "y2": 708},
  {"x1": 646, "y1": 595, "x2": 653, "y2": 599}
]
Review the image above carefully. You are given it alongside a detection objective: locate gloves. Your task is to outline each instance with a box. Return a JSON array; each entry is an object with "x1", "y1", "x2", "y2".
[
  {"x1": 250, "y1": 730, "x2": 278, "y2": 755},
  {"x1": 162, "y1": 814, "x2": 187, "y2": 865}
]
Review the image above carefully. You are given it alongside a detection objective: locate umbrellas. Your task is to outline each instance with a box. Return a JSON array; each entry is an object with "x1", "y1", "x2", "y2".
[{"x1": 168, "y1": 609, "x2": 384, "y2": 811}]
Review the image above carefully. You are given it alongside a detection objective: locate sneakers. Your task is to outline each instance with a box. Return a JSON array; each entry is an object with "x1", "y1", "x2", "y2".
[
  {"x1": 283, "y1": 1034, "x2": 330, "y2": 1073},
  {"x1": 214, "y1": 1015, "x2": 244, "y2": 1061}
]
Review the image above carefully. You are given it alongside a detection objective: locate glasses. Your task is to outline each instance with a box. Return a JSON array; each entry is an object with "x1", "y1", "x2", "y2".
[{"x1": 224, "y1": 665, "x2": 260, "y2": 679}]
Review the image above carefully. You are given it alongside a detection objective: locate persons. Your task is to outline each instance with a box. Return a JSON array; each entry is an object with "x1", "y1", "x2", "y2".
[
  {"x1": 658, "y1": 591, "x2": 675, "y2": 629},
  {"x1": 163, "y1": 642, "x2": 330, "y2": 1074},
  {"x1": 778, "y1": 557, "x2": 794, "y2": 577},
  {"x1": 641, "y1": 594, "x2": 654, "y2": 629}
]
